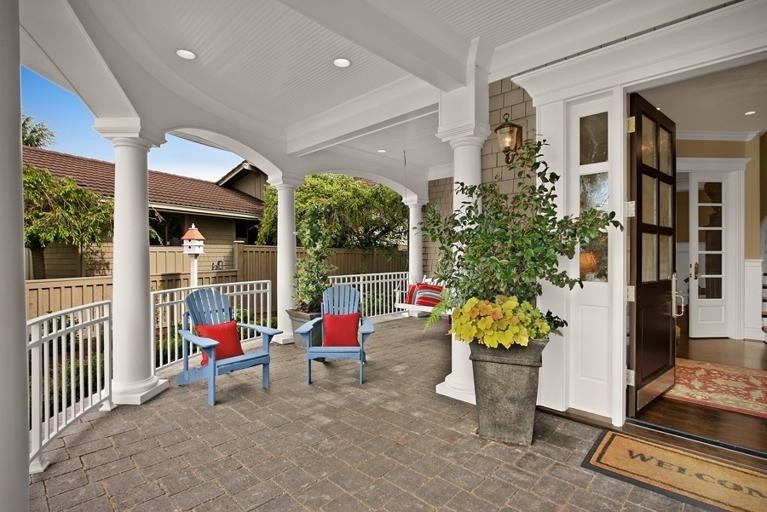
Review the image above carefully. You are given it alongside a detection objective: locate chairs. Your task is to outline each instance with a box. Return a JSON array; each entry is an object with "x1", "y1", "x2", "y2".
[
  {"x1": 294, "y1": 284, "x2": 375, "y2": 385},
  {"x1": 176, "y1": 288, "x2": 284, "y2": 406}
]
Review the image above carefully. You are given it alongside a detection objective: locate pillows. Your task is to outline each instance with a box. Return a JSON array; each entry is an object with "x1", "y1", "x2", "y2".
[
  {"x1": 405, "y1": 281, "x2": 442, "y2": 306},
  {"x1": 322, "y1": 312, "x2": 360, "y2": 346},
  {"x1": 193, "y1": 320, "x2": 245, "y2": 365}
]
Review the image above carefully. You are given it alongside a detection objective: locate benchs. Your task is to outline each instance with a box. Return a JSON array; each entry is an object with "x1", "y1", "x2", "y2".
[{"x1": 394, "y1": 274, "x2": 452, "y2": 316}]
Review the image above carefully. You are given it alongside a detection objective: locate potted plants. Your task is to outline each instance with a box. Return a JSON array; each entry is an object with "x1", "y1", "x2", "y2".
[
  {"x1": 410, "y1": 138, "x2": 625, "y2": 446},
  {"x1": 285, "y1": 239, "x2": 333, "y2": 349}
]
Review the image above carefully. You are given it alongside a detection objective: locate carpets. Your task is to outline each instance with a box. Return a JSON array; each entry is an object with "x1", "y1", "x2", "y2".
[
  {"x1": 581, "y1": 430, "x2": 767, "y2": 512},
  {"x1": 660, "y1": 357, "x2": 767, "y2": 418}
]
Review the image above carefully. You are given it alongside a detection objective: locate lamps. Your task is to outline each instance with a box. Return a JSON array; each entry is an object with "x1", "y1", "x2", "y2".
[{"x1": 494, "y1": 113, "x2": 522, "y2": 163}]
